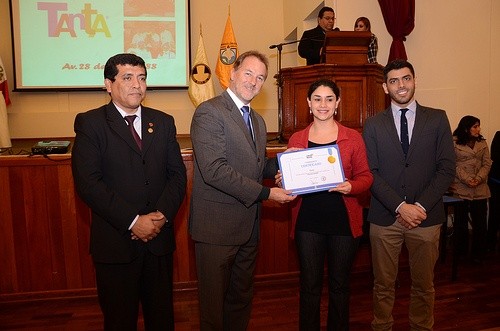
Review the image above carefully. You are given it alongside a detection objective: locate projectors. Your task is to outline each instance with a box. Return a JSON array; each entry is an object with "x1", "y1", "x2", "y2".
[{"x1": 32, "y1": 139, "x2": 71, "y2": 154}]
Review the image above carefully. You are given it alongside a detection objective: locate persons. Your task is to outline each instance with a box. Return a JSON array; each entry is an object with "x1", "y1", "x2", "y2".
[
  {"x1": 448, "y1": 115, "x2": 493, "y2": 283},
  {"x1": 487, "y1": 128, "x2": 500, "y2": 264},
  {"x1": 187, "y1": 50, "x2": 374, "y2": 331},
  {"x1": 354, "y1": 17, "x2": 379, "y2": 64},
  {"x1": 70, "y1": 53, "x2": 188, "y2": 331},
  {"x1": 298, "y1": 7, "x2": 340, "y2": 65},
  {"x1": 363, "y1": 59, "x2": 456, "y2": 331}
]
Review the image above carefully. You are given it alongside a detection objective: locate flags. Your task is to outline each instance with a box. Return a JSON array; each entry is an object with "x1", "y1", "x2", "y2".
[
  {"x1": 188, "y1": 34, "x2": 216, "y2": 108},
  {"x1": 215, "y1": 16, "x2": 240, "y2": 91}
]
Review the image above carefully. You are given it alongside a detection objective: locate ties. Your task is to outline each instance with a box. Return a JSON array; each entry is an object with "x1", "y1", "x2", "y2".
[
  {"x1": 399, "y1": 108, "x2": 409, "y2": 160},
  {"x1": 124, "y1": 115, "x2": 142, "y2": 151},
  {"x1": 241, "y1": 106, "x2": 253, "y2": 140}
]
